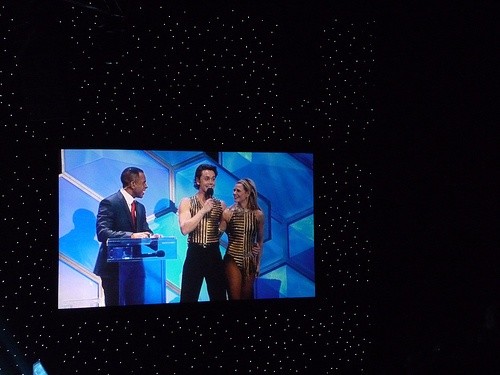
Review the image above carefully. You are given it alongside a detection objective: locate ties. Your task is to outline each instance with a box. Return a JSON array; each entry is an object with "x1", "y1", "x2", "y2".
[{"x1": 130, "y1": 200, "x2": 137, "y2": 230}]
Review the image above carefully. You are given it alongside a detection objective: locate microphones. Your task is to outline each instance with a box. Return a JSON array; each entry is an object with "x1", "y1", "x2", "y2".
[{"x1": 206, "y1": 188, "x2": 213, "y2": 217}]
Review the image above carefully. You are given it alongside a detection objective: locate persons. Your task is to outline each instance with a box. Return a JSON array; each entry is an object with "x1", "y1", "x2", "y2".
[
  {"x1": 179, "y1": 164, "x2": 261, "y2": 302},
  {"x1": 93, "y1": 167, "x2": 164, "y2": 307},
  {"x1": 186, "y1": 178, "x2": 265, "y2": 301}
]
